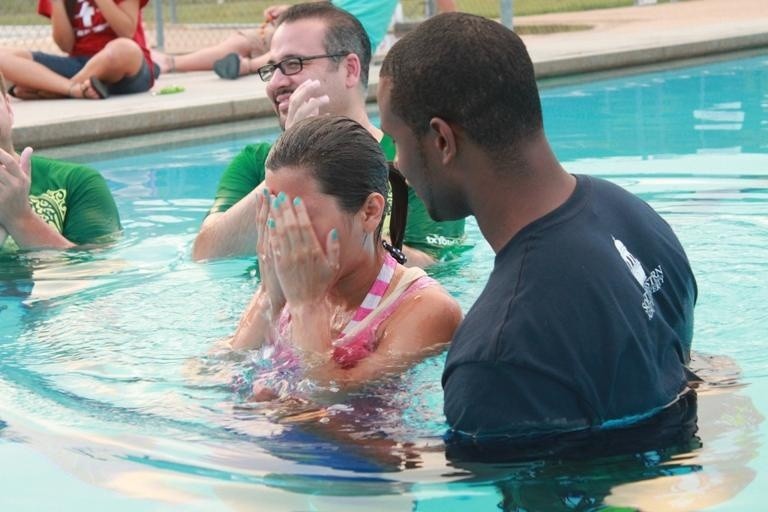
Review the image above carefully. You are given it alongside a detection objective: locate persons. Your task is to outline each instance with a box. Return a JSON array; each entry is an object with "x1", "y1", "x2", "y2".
[
  {"x1": 270, "y1": 10, "x2": 698, "y2": 466},
  {"x1": 227, "y1": 114, "x2": 463, "y2": 390},
  {"x1": 0, "y1": 70, "x2": 122, "y2": 251},
  {"x1": 146, "y1": 0, "x2": 399, "y2": 79},
  {"x1": 0, "y1": 0, "x2": 161, "y2": 100},
  {"x1": 192, "y1": 2, "x2": 465, "y2": 267}
]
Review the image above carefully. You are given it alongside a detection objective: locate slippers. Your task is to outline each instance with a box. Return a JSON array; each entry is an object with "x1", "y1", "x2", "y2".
[
  {"x1": 214, "y1": 53, "x2": 239, "y2": 79},
  {"x1": 83, "y1": 76, "x2": 110, "y2": 99},
  {"x1": 151, "y1": 62, "x2": 160, "y2": 79},
  {"x1": 8, "y1": 84, "x2": 65, "y2": 99}
]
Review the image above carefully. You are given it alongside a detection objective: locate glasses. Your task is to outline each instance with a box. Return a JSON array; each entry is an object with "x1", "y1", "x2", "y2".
[{"x1": 258, "y1": 51, "x2": 347, "y2": 82}]
